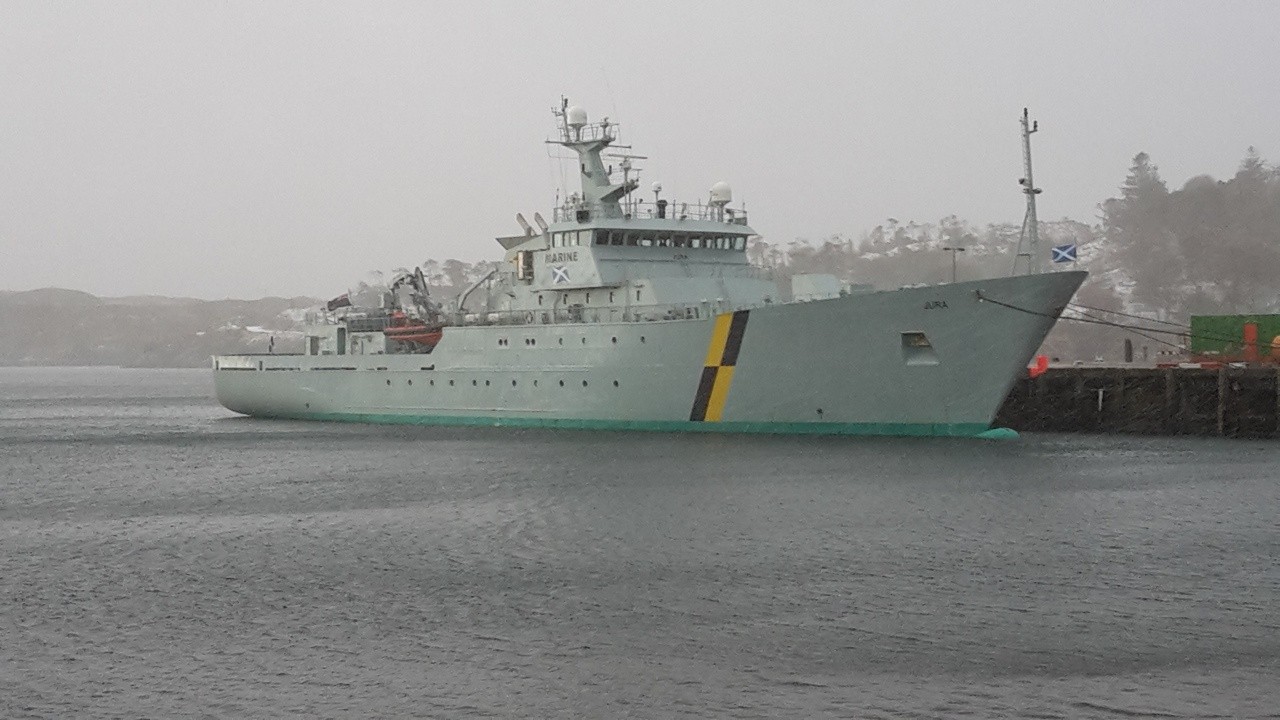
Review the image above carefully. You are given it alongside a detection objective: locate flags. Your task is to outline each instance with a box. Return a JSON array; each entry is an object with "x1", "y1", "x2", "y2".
[{"x1": 1051, "y1": 243, "x2": 1078, "y2": 263}]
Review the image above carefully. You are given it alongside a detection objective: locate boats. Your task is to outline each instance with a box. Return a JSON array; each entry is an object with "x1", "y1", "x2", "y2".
[{"x1": 212, "y1": 111, "x2": 1090, "y2": 437}]
